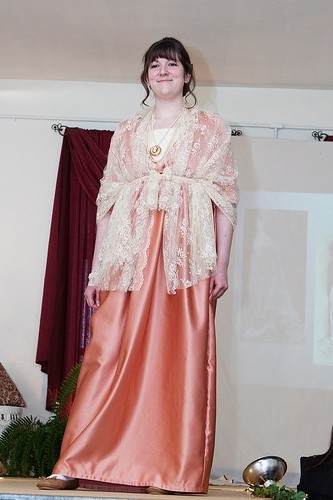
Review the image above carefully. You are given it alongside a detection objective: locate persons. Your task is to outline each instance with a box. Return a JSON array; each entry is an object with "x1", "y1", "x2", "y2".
[{"x1": 36, "y1": 37, "x2": 238, "y2": 494}]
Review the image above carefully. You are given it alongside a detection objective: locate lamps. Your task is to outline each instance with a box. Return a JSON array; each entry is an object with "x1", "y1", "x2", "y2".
[{"x1": 242, "y1": 455, "x2": 293, "y2": 497}]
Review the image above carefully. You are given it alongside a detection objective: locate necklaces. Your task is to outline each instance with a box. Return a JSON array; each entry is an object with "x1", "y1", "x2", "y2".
[{"x1": 141, "y1": 107, "x2": 184, "y2": 163}]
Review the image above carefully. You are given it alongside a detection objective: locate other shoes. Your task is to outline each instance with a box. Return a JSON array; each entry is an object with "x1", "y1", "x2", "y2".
[
  {"x1": 146, "y1": 486, "x2": 174, "y2": 495},
  {"x1": 37, "y1": 477, "x2": 80, "y2": 490}
]
